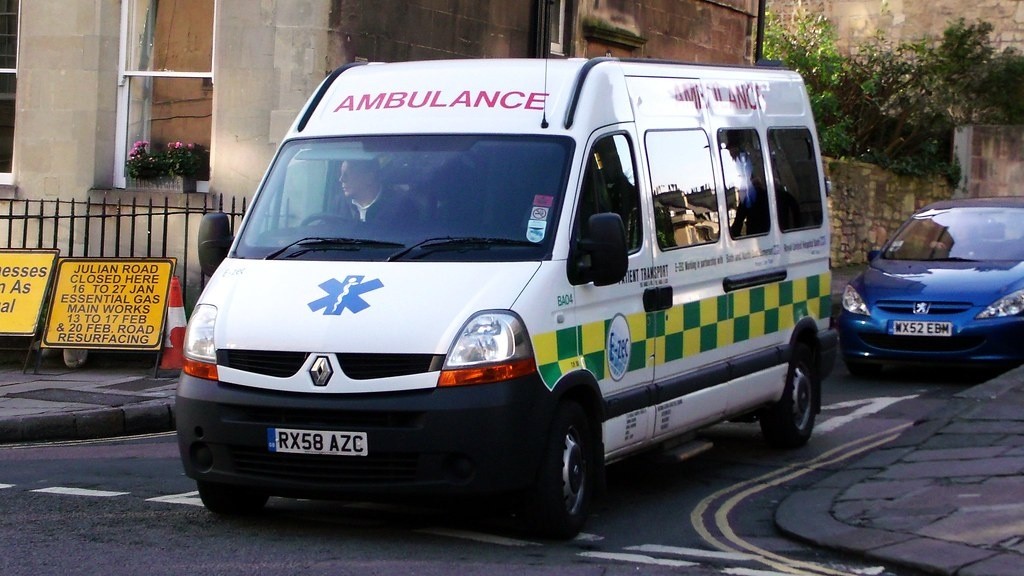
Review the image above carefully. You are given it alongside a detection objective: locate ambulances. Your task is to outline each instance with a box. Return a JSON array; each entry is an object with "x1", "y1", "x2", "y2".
[{"x1": 174, "y1": 61, "x2": 833, "y2": 542}]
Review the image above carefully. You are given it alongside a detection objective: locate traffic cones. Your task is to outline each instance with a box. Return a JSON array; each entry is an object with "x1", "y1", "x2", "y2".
[{"x1": 155, "y1": 276, "x2": 188, "y2": 379}]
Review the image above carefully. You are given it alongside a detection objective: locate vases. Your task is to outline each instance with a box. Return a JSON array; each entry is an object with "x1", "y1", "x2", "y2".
[{"x1": 141, "y1": 168, "x2": 168, "y2": 178}]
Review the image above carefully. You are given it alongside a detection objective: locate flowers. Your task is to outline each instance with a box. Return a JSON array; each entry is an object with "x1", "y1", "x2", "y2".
[{"x1": 124, "y1": 140, "x2": 209, "y2": 181}]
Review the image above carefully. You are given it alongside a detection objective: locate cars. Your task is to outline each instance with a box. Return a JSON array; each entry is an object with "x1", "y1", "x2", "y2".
[{"x1": 832, "y1": 195, "x2": 1023, "y2": 381}]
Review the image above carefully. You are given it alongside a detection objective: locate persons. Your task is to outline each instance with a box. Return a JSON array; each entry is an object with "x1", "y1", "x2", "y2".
[
  {"x1": 927, "y1": 222, "x2": 975, "y2": 250},
  {"x1": 319, "y1": 156, "x2": 418, "y2": 235}
]
